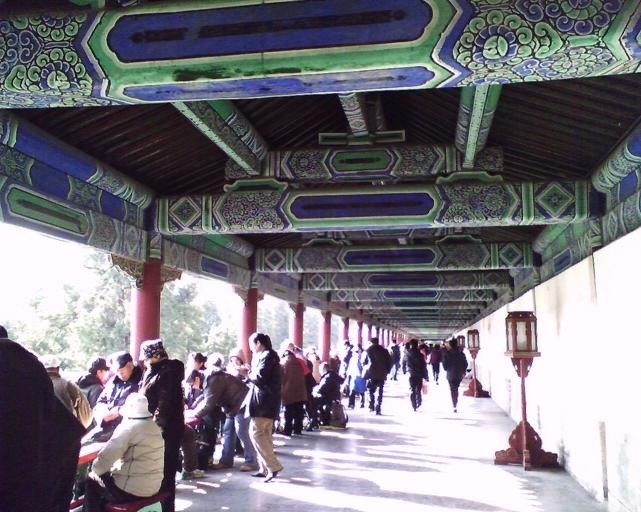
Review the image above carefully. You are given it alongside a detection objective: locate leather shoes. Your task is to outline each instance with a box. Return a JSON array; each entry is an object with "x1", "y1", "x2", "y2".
[
  {"x1": 212, "y1": 462, "x2": 232, "y2": 469},
  {"x1": 250, "y1": 472, "x2": 265, "y2": 477},
  {"x1": 240, "y1": 465, "x2": 261, "y2": 471},
  {"x1": 264, "y1": 467, "x2": 284, "y2": 482}
]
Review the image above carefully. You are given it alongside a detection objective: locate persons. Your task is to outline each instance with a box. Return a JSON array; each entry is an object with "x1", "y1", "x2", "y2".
[
  {"x1": 184, "y1": 368, "x2": 261, "y2": 471},
  {"x1": 184, "y1": 383, "x2": 222, "y2": 469},
  {"x1": 348, "y1": 344, "x2": 368, "y2": 408},
  {"x1": 429, "y1": 344, "x2": 434, "y2": 356},
  {"x1": 74, "y1": 358, "x2": 111, "y2": 501},
  {"x1": 187, "y1": 353, "x2": 208, "y2": 383},
  {"x1": 403, "y1": 339, "x2": 429, "y2": 410},
  {"x1": 361, "y1": 337, "x2": 393, "y2": 415},
  {"x1": 183, "y1": 423, "x2": 205, "y2": 479},
  {"x1": 0, "y1": 326, "x2": 87, "y2": 512},
  {"x1": 240, "y1": 333, "x2": 284, "y2": 482},
  {"x1": 387, "y1": 339, "x2": 400, "y2": 380},
  {"x1": 225, "y1": 348, "x2": 252, "y2": 458},
  {"x1": 342, "y1": 340, "x2": 354, "y2": 398},
  {"x1": 403, "y1": 343, "x2": 410, "y2": 373},
  {"x1": 279, "y1": 350, "x2": 308, "y2": 436},
  {"x1": 205, "y1": 353, "x2": 225, "y2": 369},
  {"x1": 331, "y1": 356, "x2": 341, "y2": 375},
  {"x1": 440, "y1": 344, "x2": 448, "y2": 352},
  {"x1": 418, "y1": 339, "x2": 428, "y2": 361},
  {"x1": 38, "y1": 354, "x2": 92, "y2": 428},
  {"x1": 306, "y1": 361, "x2": 345, "y2": 432},
  {"x1": 288, "y1": 343, "x2": 321, "y2": 433},
  {"x1": 80, "y1": 351, "x2": 143, "y2": 446},
  {"x1": 138, "y1": 340, "x2": 186, "y2": 512},
  {"x1": 443, "y1": 339, "x2": 469, "y2": 412},
  {"x1": 429, "y1": 344, "x2": 444, "y2": 384},
  {"x1": 306, "y1": 347, "x2": 323, "y2": 385},
  {"x1": 79, "y1": 393, "x2": 165, "y2": 512}
]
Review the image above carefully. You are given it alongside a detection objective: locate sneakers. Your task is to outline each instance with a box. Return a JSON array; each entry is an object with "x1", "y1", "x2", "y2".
[{"x1": 182, "y1": 469, "x2": 205, "y2": 480}]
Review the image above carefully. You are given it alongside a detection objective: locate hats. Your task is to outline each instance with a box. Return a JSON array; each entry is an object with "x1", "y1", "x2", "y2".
[
  {"x1": 139, "y1": 339, "x2": 165, "y2": 360},
  {"x1": 110, "y1": 351, "x2": 133, "y2": 370},
  {"x1": 118, "y1": 392, "x2": 153, "y2": 418},
  {"x1": 89, "y1": 358, "x2": 110, "y2": 372},
  {"x1": 38, "y1": 354, "x2": 62, "y2": 368}
]
